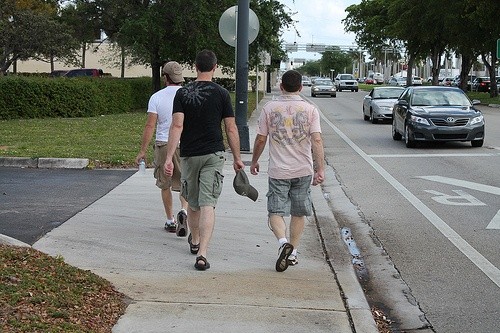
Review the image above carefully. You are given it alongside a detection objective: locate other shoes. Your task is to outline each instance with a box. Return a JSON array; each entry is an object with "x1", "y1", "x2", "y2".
[
  {"x1": 165, "y1": 221, "x2": 176, "y2": 232},
  {"x1": 176, "y1": 211, "x2": 188, "y2": 237},
  {"x1": 275, "y1": 243, "x2": 294, "y2": 272},
  {"x1": 288, "y1": 258, "x2": 299, "y2": 266}
]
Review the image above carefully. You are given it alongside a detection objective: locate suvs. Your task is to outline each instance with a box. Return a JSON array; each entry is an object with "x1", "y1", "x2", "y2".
[{"x1": 334, "y1": 74, "x2": 359, "y2": 92}]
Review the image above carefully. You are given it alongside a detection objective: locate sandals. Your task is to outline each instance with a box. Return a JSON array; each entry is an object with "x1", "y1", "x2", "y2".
[
  {"x1": 188, "y1": 233, "x2": 200, "y2": 255},
  {"x1": 194, "y1": 255, "x2": 210, "y2": 271}
]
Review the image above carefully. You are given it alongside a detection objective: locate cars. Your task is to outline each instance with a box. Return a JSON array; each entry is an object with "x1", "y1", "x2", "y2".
[
  {"x1": 358, "y1": 72, "x2": 407, "y2": 85},
  {"x1": 426, "y1": 74, "x2": 477, "y2": 91},
  {"x1": 472, "y1": 77, "x2": 491, "y2": 92},
  {"x1": 362, "y1": 87, "x2": 405, "y2": 124},
  {"x1": 301, "y1": 76, "x2": 322, "y2": 87},
  {"x1": 392, "y1": 86, "x2": 485, "y2": 147},
  {"x1": 412, "y1": 76, "x2": 423, "y2": 85},
  {"x1": 310, "y1": 78, "x2": 336, "y2": 98},
  {"x1": 496, "y1": 76, "x2": 500, "y2": 93}
]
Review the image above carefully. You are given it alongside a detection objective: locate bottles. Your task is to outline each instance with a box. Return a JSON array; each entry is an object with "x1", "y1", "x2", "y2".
[{"x1": 139, "y1": 159, "x2": 146, "y2": 175}]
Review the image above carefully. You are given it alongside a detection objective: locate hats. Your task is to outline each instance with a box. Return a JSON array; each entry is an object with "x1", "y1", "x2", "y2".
[
  {"x1": 163, "y1": 61, "x2": 185, "y2": 83},
  {"x1": 233, "y1": 169, "x2": 259, "y2": 202}
]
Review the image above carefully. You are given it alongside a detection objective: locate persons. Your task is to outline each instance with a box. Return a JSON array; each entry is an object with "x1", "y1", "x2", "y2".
[
  {"x1": 135, "y1": 61, "x2": 188, "y2": 237},
  {"x1": 164, "y1": 50, "x2": 245, "y2": 270},
  {"x1": 250, "y1": 70, "x2": 325, "y2": 272}
]
